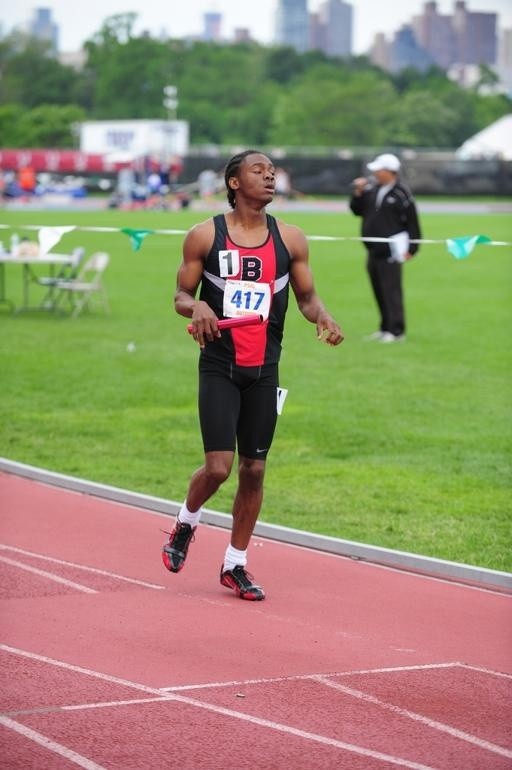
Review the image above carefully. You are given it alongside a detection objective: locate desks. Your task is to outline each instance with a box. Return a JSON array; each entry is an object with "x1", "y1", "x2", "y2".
[{"x1": 4, "y1": 251, "x2": 69, "y2": 318}]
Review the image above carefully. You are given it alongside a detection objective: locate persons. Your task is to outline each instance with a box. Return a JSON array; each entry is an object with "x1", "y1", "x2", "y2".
[
  {"x1": 162, "y1": 151, "x2": 344, "y2": 600},
  {"x1": 350, "y1": 154, "x2": 419, "y2": 342},
  {"x1": 117, "y1": 162, "x2": 289, "y2": 203}
]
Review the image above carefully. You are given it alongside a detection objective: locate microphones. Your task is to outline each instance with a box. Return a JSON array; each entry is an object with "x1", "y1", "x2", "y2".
[{"x1": 351, "y1": 176, "x2": 376, "y2": 190}]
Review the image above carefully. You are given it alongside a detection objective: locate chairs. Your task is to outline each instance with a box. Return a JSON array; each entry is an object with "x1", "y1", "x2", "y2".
[{"x1": 34, "y1": 247, "x2": 109, "y2": 317}]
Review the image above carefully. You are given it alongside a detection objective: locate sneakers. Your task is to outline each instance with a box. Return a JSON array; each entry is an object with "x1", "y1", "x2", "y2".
[
  {"x1": 220, "y1": 561, "x2": 268, "y2": 601},
  {"x1": 362, "y1": 331, "x2": 406, "y2": 346},
  {"x1": 158, "y1": 511, "x2": 199, "y2": 575}
]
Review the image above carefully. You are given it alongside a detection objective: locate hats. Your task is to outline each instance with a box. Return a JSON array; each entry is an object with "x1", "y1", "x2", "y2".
[{"x1": 366, "y1": 153, "x2": 400, "y2": 175}]
